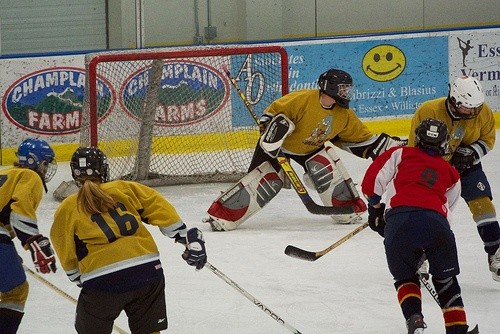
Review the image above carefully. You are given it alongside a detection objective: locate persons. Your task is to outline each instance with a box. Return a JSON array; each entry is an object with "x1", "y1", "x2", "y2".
[
  {"x1": 0, "y1": 138, "x2": 57, "y2": 334},
  {"x1": 361, "y1": 117, "x2": 479, "y2": 334},
  {"x1": 203, "y1": 68, "x2": 408, "y2": 231},
  {"x1": 408, "y1": 75, "x2": 500, "y2": 277},
  {"x1": 50, "y1": 145, "x2": 207, "y2": 334}
]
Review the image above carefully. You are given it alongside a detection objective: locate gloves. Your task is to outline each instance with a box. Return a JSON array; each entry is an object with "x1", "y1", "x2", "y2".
[
  {"x1": 368, "y1": 203, "x2": 386, "y2": 237},
  {"x1": 29, "y1": 237, "x2": 57, "y2": 274},
  {"x1": 182, "y1": 228, "x2": 207, "y2": 270},
  {"x1": 448, "y1": 143, "x2": 475, "y2": 173}
]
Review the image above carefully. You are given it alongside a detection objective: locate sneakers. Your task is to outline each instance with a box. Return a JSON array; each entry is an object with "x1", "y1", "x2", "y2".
[
  {"x1": 405, "y1": 312, "x2": 427, "y2": 334},
  {"x1": 488, "y1": 246, "x2": 500, "y2": 282}
]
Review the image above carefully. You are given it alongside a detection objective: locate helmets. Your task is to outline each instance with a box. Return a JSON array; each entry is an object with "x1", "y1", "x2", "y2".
[
  {"x1": 415, "y1": 118, "x2": 451, "y2": 157},
  {"x1": 18, "y1": 137, "x2": 58, "y2": 183},
  {"x1": 448, "y1": 75, "x2": 485, "y2": 120},
  {"x1": 318, "y1": 68, "x2": 353, "y2": 110},
  {"x1": 70, "y1": 146, "x2": 111, "y2": 188}
]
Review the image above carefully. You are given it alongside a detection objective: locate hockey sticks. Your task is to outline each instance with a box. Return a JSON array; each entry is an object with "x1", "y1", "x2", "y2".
[
  {"x1": 417, "y1": 270, "x2": 480, "y2": 334},
  {"x1": 284, "y1": 221, "x2": 369, "y2": 262},
  {"x1": 220, "y1": 65, "x2": 354, "y2": 215},
  {"x1": 205, "y1": 262, "x2": 303, "y2": 334},
  {"x1": 22, "y1": 264, "x2": 128, "y2": 334}
]
